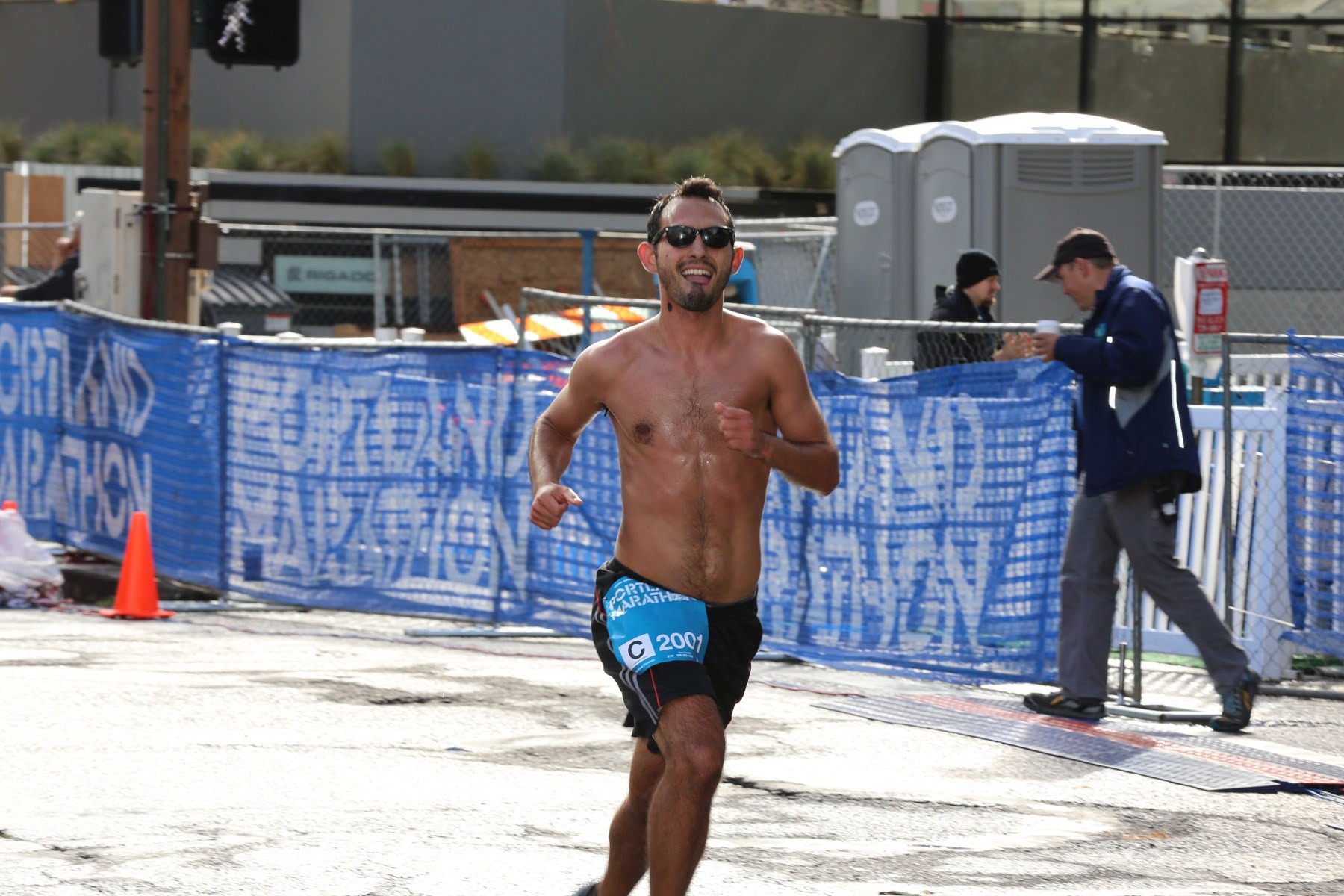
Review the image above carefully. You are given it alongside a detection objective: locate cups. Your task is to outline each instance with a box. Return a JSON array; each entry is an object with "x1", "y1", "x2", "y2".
[
  {"x1": 400, "y1": 327, "x2": 426, "y2": 342},
  {"x1": 860, "y1": 346, "x2": 889, "y2": 379},
  {"x1": 216, "y1": 321, "x2": 243, "y2": 336},
  {"x1": 1036, "y1": 319, "x2": 1060, "y2": 334},
  {"x1": 884, "y1": 361, "x2": 914, "y2": 378},
  {"x1": 374, "y1": 327, "x2": 397, "y2": 341}
]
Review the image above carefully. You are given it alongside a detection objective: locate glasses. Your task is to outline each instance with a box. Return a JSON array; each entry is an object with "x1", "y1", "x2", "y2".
[{"x1": 650, "y1": 225, "x2": 733, "y2": 249}]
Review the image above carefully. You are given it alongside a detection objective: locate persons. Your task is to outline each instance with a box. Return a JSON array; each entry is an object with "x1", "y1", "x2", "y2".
[
  {"x1": 1021, "y1": 227, "x2": 1260, "y2": 733},
  {"x1": 0, "y1": 209, "x2": 83, "y2": 300},
  {"x1": 912, "y1": 247, "x2": 1034, "y2": 377},
  {"x1": 525, "y1": 175, "x2": 842, "y2": 896}
]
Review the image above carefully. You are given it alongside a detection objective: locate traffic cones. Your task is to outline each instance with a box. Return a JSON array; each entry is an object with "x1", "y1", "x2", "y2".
[{"x1": 98, "y1": 512, "x2": 175, "y2": 619}]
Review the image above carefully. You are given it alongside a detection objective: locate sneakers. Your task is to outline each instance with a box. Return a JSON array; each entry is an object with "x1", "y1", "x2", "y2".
[
  {"x1": 1023, "y1": 691, "x2": 1106, "y2": 720},
  {"x1": 1210, "y1": 668, "x2": 1261, "y2": 730}
]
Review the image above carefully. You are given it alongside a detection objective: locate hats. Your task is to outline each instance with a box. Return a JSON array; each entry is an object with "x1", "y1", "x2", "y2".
[
  {"x1": 1033, "y1": 227, "x2": 1117, "y2": 280},
  {"x1": 956, "y1": 251, "x2": 999, "y2": 288}
]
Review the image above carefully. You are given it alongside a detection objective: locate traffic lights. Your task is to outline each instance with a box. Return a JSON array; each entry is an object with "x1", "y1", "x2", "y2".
[{"x1": 204, "y1": 0, "x2": 301, "y2": 71}]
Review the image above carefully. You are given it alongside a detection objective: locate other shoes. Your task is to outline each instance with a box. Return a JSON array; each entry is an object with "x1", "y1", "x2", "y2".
[{"x1": 575, "y1": 883, "x2": 599, "y2": 896}]
